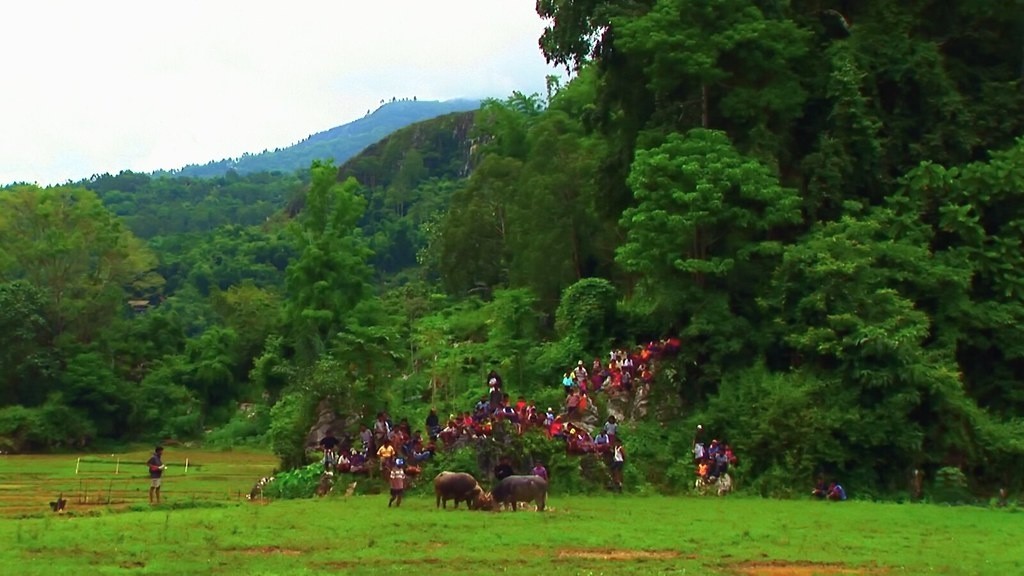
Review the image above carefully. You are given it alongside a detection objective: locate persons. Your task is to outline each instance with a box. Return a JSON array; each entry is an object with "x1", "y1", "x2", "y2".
[
  {"x1": 147, "y1": 447, "x2": 166, "y2": 505},
  {"x1": 812, "y1": 475, "x2": 846, "y2": 502},
  {"x1": 319, "y1": 407, "x2": 439, "y2": 507},
  {"x1": 562, "y1": 337, "x2": 680, "y2": 486},
  {"x1": 692, "y1": 424, "x2": 735, "y2": 496},
  {"x1": 533, "y1": 459, "x2": 549, "y2": 482},
  {"x1": 493, "y1": 456, "x2": 518, "y2": 482},
  {"x1": 439, "y1": 370, "x2": 564, "y2": 444}
]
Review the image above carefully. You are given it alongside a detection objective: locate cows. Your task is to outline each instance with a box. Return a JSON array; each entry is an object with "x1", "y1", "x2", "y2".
[{"x1": 434, "y1": 471, "x2": 549, "y2": 513}]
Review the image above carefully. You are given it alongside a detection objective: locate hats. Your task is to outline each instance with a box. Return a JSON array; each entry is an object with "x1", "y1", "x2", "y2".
[
  {"x1": 712, "y1": 439, "x2": 717, "y2": 443},
  {"x1": 697, "y1": 424, "x2": 702, "y2": 430},
  {"x1": 395, "y1": 458, "x2": 404, "y2": 466},
  {"x1": 547, "y1": 407, "x2": 553, "y2": 412},
  {"x1": 578, "y1": 360, "x2": 583, "y2": 365}
]
